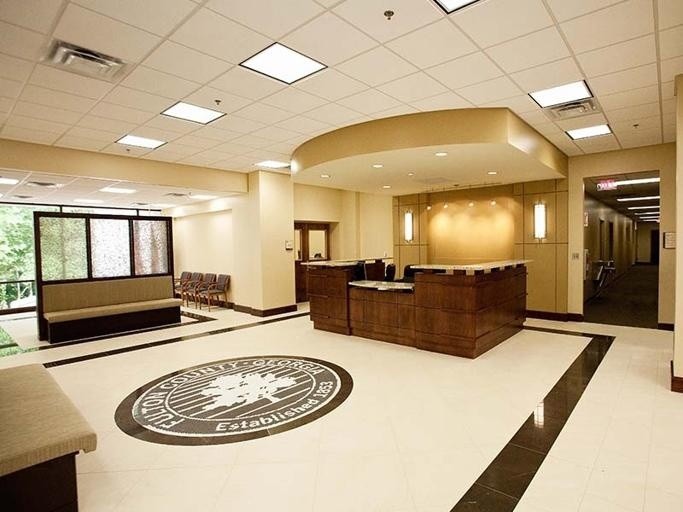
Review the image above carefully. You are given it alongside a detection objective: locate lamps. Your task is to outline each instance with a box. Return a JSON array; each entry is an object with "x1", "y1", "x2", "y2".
[
  {"x1": 403, "y1": 208, "x2": 414, "y2": 243},
  {"x1": 533, "y1": 199, "x2": 546, "y2": 243}
]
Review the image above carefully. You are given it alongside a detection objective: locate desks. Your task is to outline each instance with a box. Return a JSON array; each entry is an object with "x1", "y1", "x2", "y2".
[{"x1": 0, "y1": 363, "x2": 97, "y2": 512}]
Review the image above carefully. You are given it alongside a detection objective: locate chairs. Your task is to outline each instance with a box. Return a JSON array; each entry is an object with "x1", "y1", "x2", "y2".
[
  {"x1": 383, "y1": 264, "x2": 397, "y2": 282},
  {"x1": 173, "y1": 272, "x2": 230, "y2": 312},
  {"x1": 395, "y1": 264, "x2": 422, "y2": 283}
]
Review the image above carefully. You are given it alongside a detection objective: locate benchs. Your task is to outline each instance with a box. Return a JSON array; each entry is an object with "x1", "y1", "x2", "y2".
[{"x1": 41, "y1": 274, "x2": 183, "y2": 344}]
